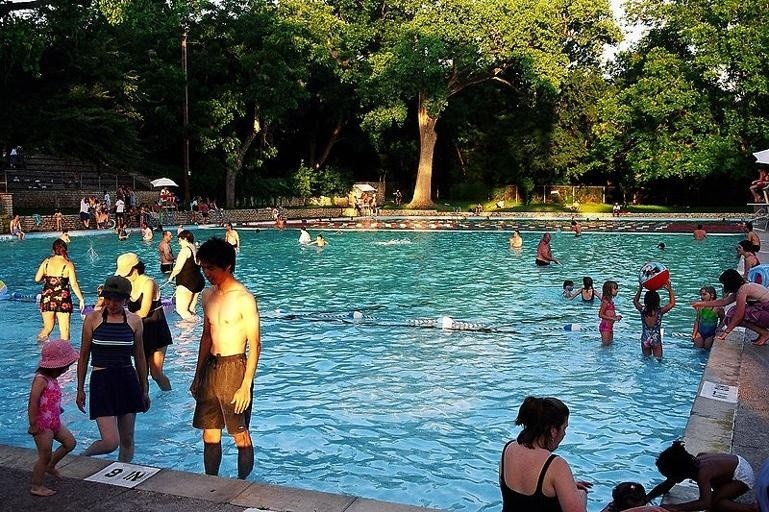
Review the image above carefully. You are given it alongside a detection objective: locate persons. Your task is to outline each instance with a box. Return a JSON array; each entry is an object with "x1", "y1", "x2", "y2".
[
  {"x1": 168, "y1": 230, "x2": 206, "y2": 322},
  {"x1": 737, "y1": 240, "x2": 762, "y2": 285},
  {"x1": 177, "y1": 225, "x2": 185, "y2": 235},
  {"x1": 656, "y1": 242, "x2": 665, "y2": 251},
  {"x1": 10, "y1": 215, "x2": 25, "y2": 240},
  {"x1": 633, "y1": 280, "x2": 675, "y2": 357},
  {"x1": 190, "y1": 235, "x2": 261, "y2": 480},
  {"x1": 353, "y1": 191, "x2": 378, "y2": 215},
  {"x1": 60, "y1": 228, "x2": 71, "y2": 243},
  {"x1": 298, "y1": 227, "x2": 311, "y2": 244},
  {"x1": 535, "y1": 233, "x2": 560, "y2": 266},
  {"x1": 572, "y1": 200, "x2": 580, "y2": 211},
  {"x1": 621, "y1": 200, "x2": 629, "y2": 217},
  {"x1": 114, "y1": 253, "x2": 173, "y2": 391},
  {"x1": 159, "y1": 230, "x2": 174, "y2": 274},
  {"x1": 189, "y1": 196, "x2": 226, "y2": 228},
  {"x1": 35, "y1": 238, "x2": 84, "y2": 341},
  {"x1": 749, "y1": 167, "x2": 766, "y2": 203},
  {"x1": 600, "y1": 481, "x2": 669, "y2": 512},
  {"x1": 475, "y1": 203, "x2": 483, "y2": 214},
  {"x1": 743, "y1": 222, "x2": 761, "y2": 253},
  {"x1": 271, "y1": 206, "x2": 280, "y2": 221},
  {"x1": 152, "y1": 186, "x2": 181, "y2": 212},
  {"x1": 508, "y1": 230, "x2": 523, "y2": 248},
  {"x1": 613, "y1": 203, "x2": 620, "y2": 217},
  {"x1": 500, "y1": 396, "x2": 593, "y2": 511},
  {"x1": 224, "y1": 222, "x2": 240, "y2": 254},
  {"x1": 646, "y1": 440, "x2": 759, "y2": 512},
  {"x1": 691, "y1": 269, "x2": 769, "y2": 346},
  {"x1": 690, "y1": 286, "x2": 725, "y2": 348},
  {"x1": 94, "y1": 284, "x2": 105, "y2": 311},
  {"x1": 276, "y1": 214, "x2": 288, "y2": 229},
  {"x1": 562, "y1": 280, "x2": 574, "y2": 298},
  {"x1": 395, "y1": 190, "x2": 402, "y2": 206},
  {"x1": 693, "y1": 224, "x2": 707, "y2": 240},
  {"x1": 568, "y1": 277, "x2": 602, "y2": 302},
  {"x1": 598, "y1": 281, "x2": 623, "y2": 345},
  {"x1": 571, "y1": 220, "x2": 582, "y2": 238},
  {"x1": 76, "y1": 275, "x2": 152, "y2": 463},
  {"x1": 79, "y1": 185, "x2": 154, "y2": 241},
  {"x1": 308, "y1": 234, "x2": 329, "y2": 247},
  {"x1": 55, "y1": 209, "x2": 64, "y2": 231},
  {"x1": 28, "y1": 339, "x2": 80, "y2": 496}
]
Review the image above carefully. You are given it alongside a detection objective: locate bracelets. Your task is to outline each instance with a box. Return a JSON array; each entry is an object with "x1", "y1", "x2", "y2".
[{"x1": 77, "y1": 386, "x2": 85, "y2": 390}]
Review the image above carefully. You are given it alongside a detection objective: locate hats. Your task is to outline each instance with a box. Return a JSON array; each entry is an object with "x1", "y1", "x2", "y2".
[
  {"x1": 38, "y1": 340, "x2": 80, "y2": 368},
  {"x1": 99, "y1": 275, "x2": 132, "y2": 299},
  {"x1": 113, "y1": 253, "x2": 140, "y2": 277}
]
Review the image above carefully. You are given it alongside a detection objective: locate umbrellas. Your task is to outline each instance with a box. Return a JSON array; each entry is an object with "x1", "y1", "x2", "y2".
[{"x1": 150, "y1": 177, "x2": 180, "y2": 188}]
